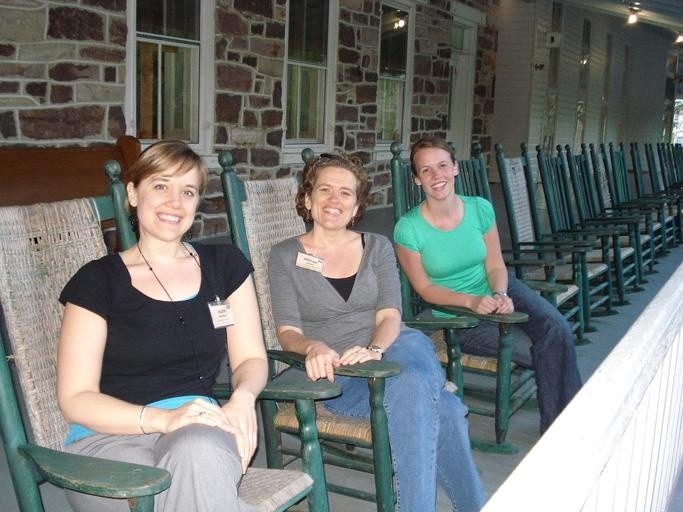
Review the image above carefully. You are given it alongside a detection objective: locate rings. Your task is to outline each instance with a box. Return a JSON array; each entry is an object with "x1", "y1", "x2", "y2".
[{"x1": 198, "y1": 410, "x2": 206, "y2": 417}]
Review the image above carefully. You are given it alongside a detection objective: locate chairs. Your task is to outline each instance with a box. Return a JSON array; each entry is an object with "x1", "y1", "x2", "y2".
[
  {"x1": 1, "y1": 161, "x2": 343, "y2": 512},
  {"x1": 491, "y1": 141, "x2": 683, "y2": 348},
  {"x1": 218, "y1": 148, "x2": 481, "y2": 512}
]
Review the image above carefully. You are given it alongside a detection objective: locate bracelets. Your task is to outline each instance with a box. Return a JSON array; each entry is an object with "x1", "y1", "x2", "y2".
[{"x1": 138, "y1": 405, "x2": 148, "y2": 435}]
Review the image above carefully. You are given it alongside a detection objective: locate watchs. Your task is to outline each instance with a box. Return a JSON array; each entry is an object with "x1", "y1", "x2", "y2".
[
  {"x1": 492, "y1": 291, "x2": 507, "y2": 298},
  {"x1": 366, "y1": 344, "x2": 385, "y2": 360}
]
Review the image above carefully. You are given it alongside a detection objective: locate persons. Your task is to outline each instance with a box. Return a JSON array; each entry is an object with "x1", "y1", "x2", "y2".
[
  {"x1": 268, "y1": 151, "x2": 485, "y2": 512},
  {"x1": 56, "y1": 138, "x2": 269, "y2": 512}
]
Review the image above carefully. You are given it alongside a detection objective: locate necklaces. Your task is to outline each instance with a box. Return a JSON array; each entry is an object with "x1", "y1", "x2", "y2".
[{"x1": 137, "y1": 239, "x2": 231, "y2": 405}]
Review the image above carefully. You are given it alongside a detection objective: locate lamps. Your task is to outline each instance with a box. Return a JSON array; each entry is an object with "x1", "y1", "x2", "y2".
[
  {"x1": 391, "y1": 9, "x2": 408, "y2": 32},
  {"x1": 626, "y1": 3, "x2": 641, "y2": 25},
  {"x1": 580, "y1": 37, "x2": 589, "y2": 65}
]
